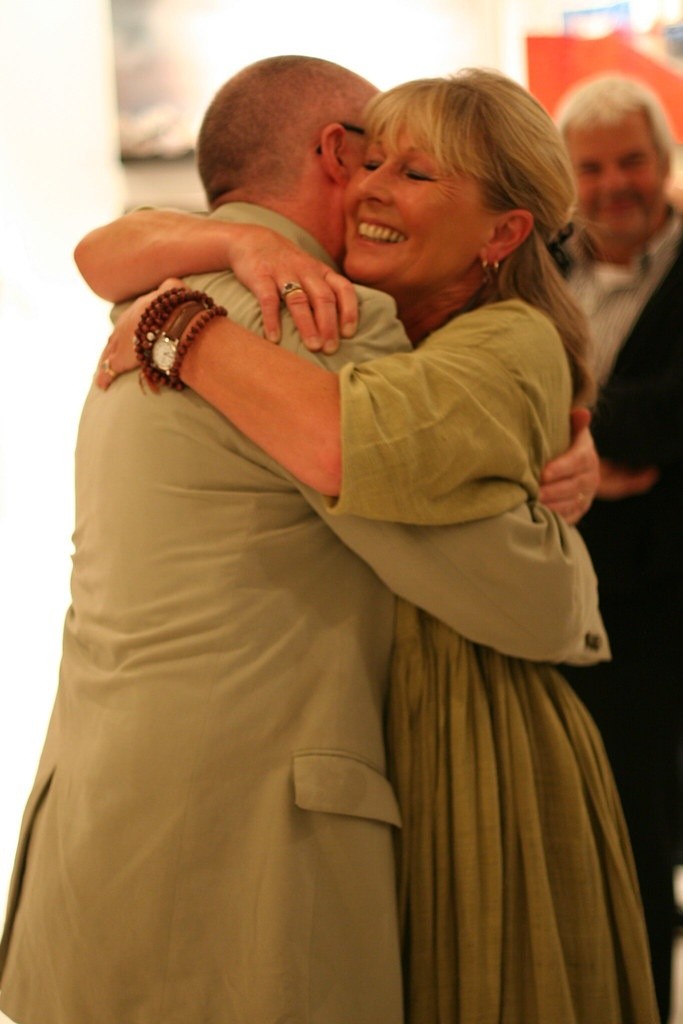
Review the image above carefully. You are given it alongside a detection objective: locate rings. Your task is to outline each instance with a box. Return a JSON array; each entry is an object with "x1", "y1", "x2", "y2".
[
  {"x1": 281, "y1": 282, "x2": 303, "y2": 302},
  {"x1": 102, "y1": 357, "x2": 118, "y2": 378}
]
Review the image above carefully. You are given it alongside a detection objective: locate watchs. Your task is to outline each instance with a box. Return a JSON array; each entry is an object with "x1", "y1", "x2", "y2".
[{"x1": 151, "y1": 303, "x2": 207, "y2": 377}]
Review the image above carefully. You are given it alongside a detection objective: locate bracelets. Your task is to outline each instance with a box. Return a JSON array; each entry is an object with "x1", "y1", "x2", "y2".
[{"x1": 133, "y1": 288, "x2": 228, "y2": 391}]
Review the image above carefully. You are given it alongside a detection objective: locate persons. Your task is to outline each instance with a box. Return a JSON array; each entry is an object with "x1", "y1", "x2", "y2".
[
  {"x1": 0, "y1": 57, "x2": 611, "y2": 1024},
  {"x1": 74, "y1": 72, "x2": 663, "y2": 1024},
  {"x1": 557, "y1": 75, "x2": 683, "y2": 1024}
]
